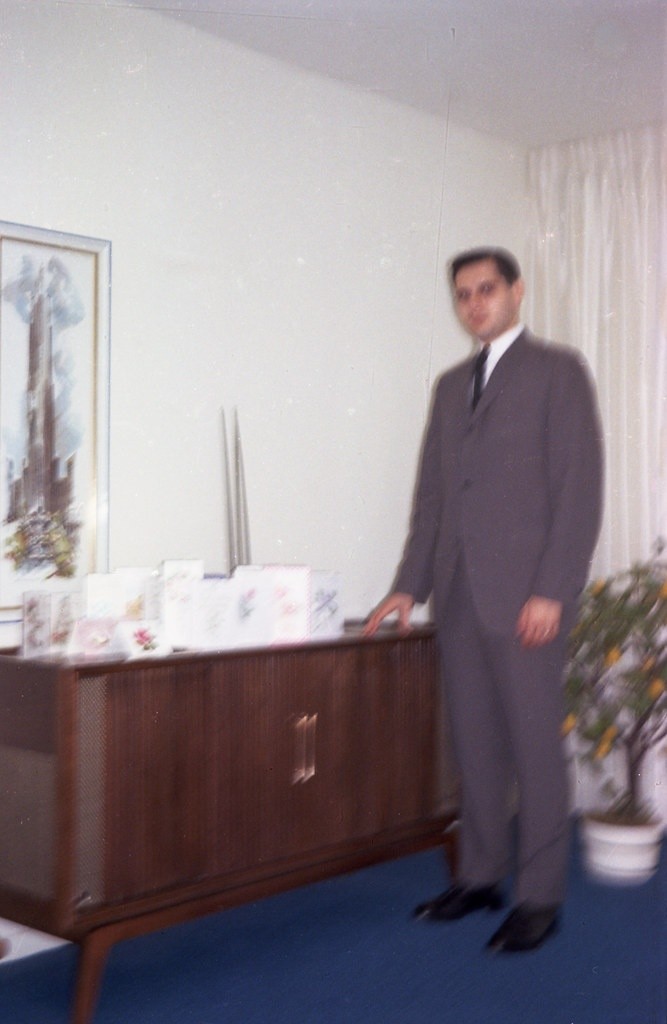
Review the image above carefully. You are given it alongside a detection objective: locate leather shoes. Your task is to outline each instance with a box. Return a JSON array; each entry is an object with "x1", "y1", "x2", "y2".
[
  {"x1": 412, "y1": 883, "x2": 503, "y2": 921},
  {"x1": 486, "y1": 906, "x2": 559, "y2": 952}
]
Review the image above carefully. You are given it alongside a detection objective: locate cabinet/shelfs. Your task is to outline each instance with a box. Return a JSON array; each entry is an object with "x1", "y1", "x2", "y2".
[{"x1": 0, "y1": 618, "x2": 460, "y2": 1024}]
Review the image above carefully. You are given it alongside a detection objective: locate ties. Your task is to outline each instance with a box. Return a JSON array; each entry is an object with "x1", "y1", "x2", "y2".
[{"x1": 470, "y1": 344, "x2": 490, "y2": 413}]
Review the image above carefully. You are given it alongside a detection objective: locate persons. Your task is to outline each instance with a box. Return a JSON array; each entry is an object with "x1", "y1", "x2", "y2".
[{"x1": 365, "y1": 250, "x2": 603, "y2": 958}]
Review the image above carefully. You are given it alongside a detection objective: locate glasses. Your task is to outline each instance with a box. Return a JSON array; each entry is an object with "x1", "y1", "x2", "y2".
[{"x1": 454, "y1": 276, "x2": 503, "y2": 300}]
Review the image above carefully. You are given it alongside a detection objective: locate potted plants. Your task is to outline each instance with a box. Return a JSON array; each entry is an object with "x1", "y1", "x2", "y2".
[{"x1": 562, "y1": 535, "x2": 667, "y2": 886}]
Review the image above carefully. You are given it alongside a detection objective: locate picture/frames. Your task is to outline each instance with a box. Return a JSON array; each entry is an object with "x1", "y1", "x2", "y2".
[{"x1": 0, "y1": 220, "x2": 111, "y2": 619}]
